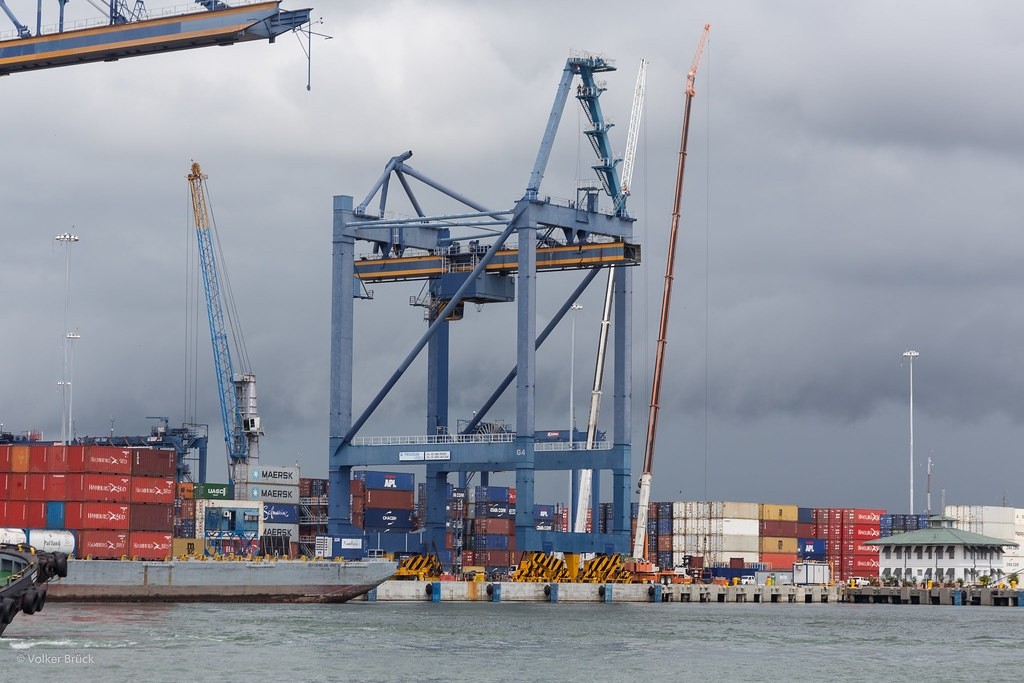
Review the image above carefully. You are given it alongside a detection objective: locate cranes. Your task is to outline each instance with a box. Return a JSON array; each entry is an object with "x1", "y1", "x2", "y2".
[{"x1": 175, "y1": 160, "x2": 267, "y2": 498}]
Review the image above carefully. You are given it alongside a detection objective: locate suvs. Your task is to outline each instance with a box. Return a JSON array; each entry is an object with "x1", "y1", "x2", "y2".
[{"x1": 847, "y1": 577, "x2": 871, "y2": 585}]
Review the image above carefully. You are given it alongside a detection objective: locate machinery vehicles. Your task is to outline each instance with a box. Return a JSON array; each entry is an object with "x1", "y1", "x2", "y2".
[
  {"x1": 551, "y1": 62, "x2": 651, "y2": 570},
  {"x1": 625, "y1": 23, "x2": 718, "y2": 585}
]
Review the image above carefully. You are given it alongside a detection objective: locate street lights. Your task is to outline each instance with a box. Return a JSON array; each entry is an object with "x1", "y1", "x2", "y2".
[
  {"x1": 57, "y1": 381, "x2": 71, "y2": 440},
  {"x1": 903, "y1": 349, "x2": 920, "y2": 514},
  {"x1": 55, "y1": 232, "x2": 79, "y2": 446},
  {"x1": 569, "y1": 304, "x2": 583, "y2": 532},
  {"x1": 66, "y1": 333, "x2": 80, "y2": 445}
]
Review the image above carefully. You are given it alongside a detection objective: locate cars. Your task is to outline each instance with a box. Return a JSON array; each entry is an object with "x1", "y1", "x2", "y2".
[{"x1": 741, "y1": 575, "x2": 756, "y2": 584}]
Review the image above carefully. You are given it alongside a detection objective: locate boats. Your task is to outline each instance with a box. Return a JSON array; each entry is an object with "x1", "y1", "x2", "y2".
[
  {"x1": 0, "y1": 541, "x2": 67, "y2": 638},
  {"x1": 43, "y1": 561, "x2": 399, "y2": 603}
]
